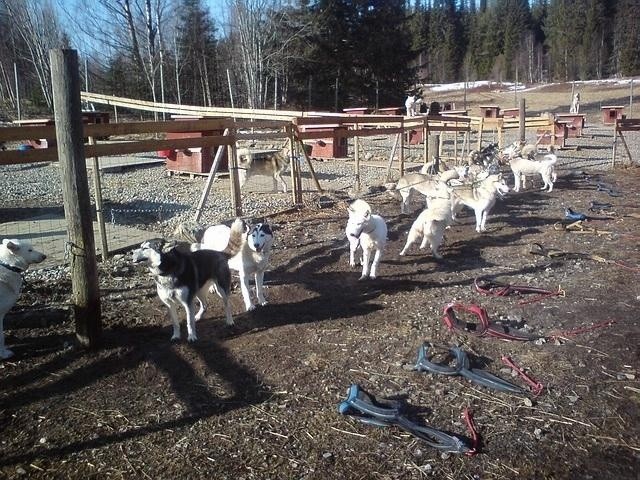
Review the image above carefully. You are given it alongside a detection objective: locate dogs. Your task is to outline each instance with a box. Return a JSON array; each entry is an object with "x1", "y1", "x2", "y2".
[
  {"x1": 405, "y1": 95, "x2": 415, "y2": 118},
  {"x1": 345, "y1": 199, "x2": 388, "y2": 281},
  {"x1": 395, "y1": 139, "x2": 559, "y2": 259},
  {"x1": 0, "y1": 238, "x2": 48, "y2": 359},
  {"x1": 132, "y1": 216, "x2": 244, "y2": 344},
  {"x1": 237, "y1": 146, "x2": 302, "y2": 195},
  {"x1": 569, "y1": 92, "x2": 580, "y2": 114},
  {"x1": 191, "y1": 215, "x2": 274, "y2": 312}
]
